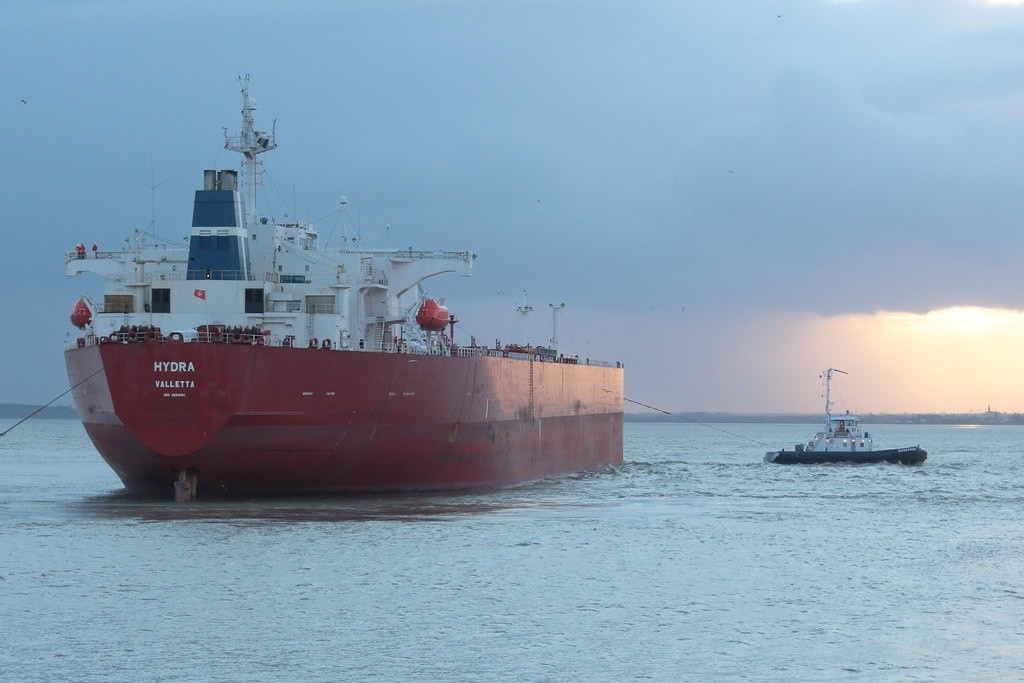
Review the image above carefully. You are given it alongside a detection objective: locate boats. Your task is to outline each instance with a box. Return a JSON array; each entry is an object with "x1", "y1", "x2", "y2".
[
  {"x1": 759, "y1": 368, "x2": 929, "y2": 466},
  {"x1": 63, "y1": 68, "x2": 626, "y2": 504}
]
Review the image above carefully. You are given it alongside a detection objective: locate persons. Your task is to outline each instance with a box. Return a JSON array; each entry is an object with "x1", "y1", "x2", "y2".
[
  {"x1": 75, "y1": 242, "x2": 86, "y2": 260},
  {"x1": 92, "y1": 243, "x2": 98, "y2": 259}
]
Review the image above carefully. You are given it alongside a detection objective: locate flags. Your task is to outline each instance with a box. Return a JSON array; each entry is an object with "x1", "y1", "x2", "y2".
[{"x1": 194, "y1": 289, "x2": 205, "y2": 300}]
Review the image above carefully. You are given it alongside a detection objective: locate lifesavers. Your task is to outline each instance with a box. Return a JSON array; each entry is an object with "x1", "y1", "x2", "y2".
[
  {"x1": 168, "y1": 332, "x2": 184, "y2": 344},
  {"x1": 322, "y1": 338, "x2": 331, "y2": 350},
  {"x1": 231, "y1": 333, "x2": 242, "y2": 344},
  {"x1": 108, "y1": 333, "x2": 120, "y2": 344},
  {"x1": 76, "y1": 338, "x2": 85, "y2": 348},
  {"x1": 257, "y1": 337, "x2": 264, "y2": 345},
  {"x1": 309, "y1": 338, "x2": 319, "y2": 349},
  {"x1": 144, "y1": 331, "x2": 157, "y2": 343},
  {"x1": 127, "y1": 333, "x2": 138, "y2": 343},
  {"x1": 100, "y1": 336, "x2": 109, "y2": 344},
  {"x1": 242, "y1": 336, "x2": 251, "y2": 344},
  {"x1": 212, "y1": 333, "x2": 224, "y2": 343}
]
